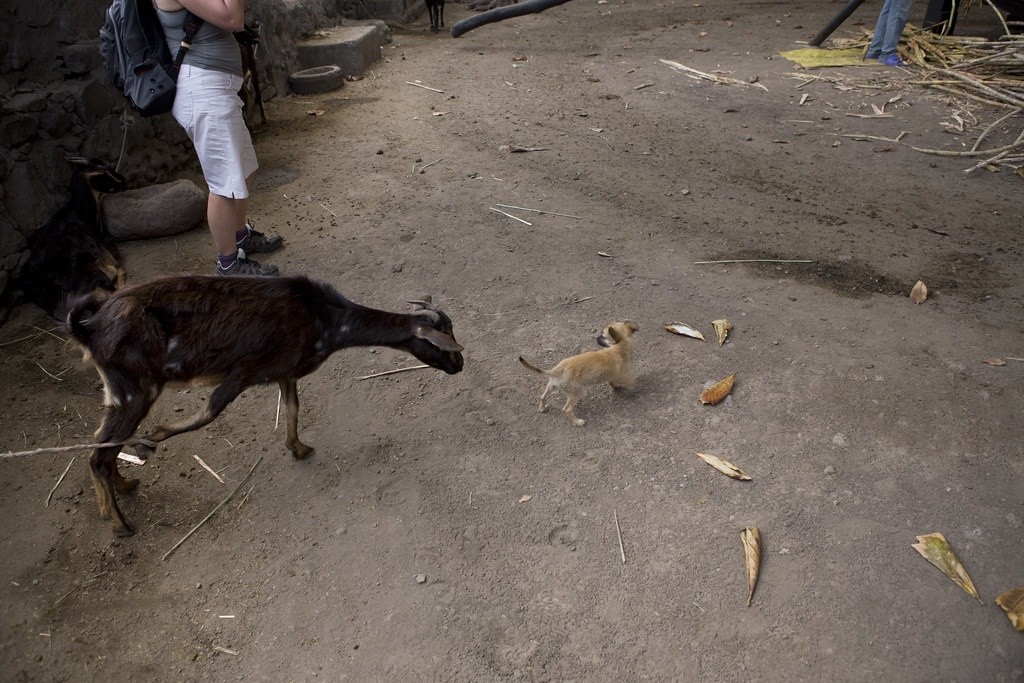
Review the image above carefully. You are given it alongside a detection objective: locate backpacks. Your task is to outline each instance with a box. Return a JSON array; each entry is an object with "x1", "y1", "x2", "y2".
[{"x1": 99, "y1": 0, "x2": 204, "y2": 118}]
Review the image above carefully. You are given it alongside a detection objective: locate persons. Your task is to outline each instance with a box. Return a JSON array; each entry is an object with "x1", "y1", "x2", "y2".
[
  {"x1": 864, "y1": 0, "x2": 914, "y2": 68},
  {"x1": 152, "y1": 1, "x2": 285, "y2": 278}
]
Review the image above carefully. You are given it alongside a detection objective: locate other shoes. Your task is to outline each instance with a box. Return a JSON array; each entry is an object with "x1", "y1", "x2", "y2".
[{"x1": 879, "y1": 54, "x2": 909, "y2": 65}]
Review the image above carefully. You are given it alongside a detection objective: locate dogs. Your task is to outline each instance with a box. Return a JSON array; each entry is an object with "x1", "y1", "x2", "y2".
[{"x1": 518, "y1": 320, "x2": 638, "y2": 426}]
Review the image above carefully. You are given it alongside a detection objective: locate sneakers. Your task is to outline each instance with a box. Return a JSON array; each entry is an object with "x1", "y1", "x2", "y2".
[
  {"x1": 215, "y1": 248, "x2": 279, "y2": 276},
  {"x1": 236, "y1": 219, "x2": 283, "y2": 255}
]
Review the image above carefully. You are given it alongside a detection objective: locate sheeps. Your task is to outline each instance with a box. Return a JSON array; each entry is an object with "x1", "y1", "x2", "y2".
[{"x1": 62, "y1": 273, "x2": 464, "y2": 537}]
